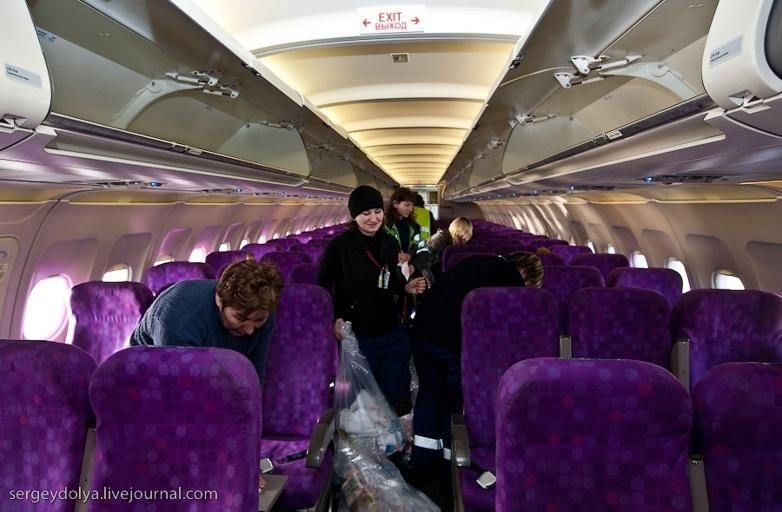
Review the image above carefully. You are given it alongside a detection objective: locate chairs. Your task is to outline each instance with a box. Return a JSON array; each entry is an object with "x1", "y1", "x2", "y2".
[
  {"x1": 1, "y1": 221, "x2": 355, "y2": 512},
  {"x1": 439, "y1": 220, "x2": 781, "y2": 511}
]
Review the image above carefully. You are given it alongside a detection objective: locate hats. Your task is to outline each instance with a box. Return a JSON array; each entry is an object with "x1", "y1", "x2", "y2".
[{"x1": 348, "y1": 186, "x2": 384, "y2": 219}]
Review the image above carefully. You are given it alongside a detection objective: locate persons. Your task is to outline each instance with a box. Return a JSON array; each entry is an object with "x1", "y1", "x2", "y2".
[{"x1": 128, "y1": 262, "x2": 285, "y2": 489}]
